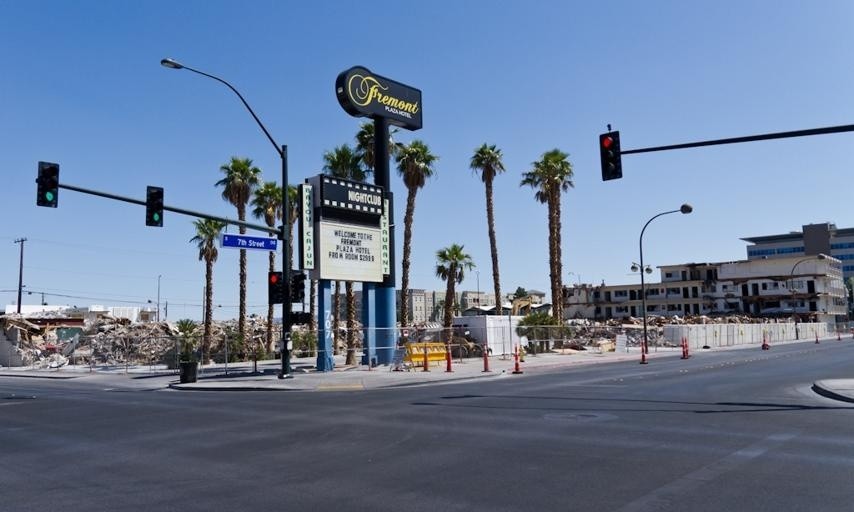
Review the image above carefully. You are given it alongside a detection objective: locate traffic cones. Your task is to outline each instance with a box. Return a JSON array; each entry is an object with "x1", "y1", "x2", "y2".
[
  {"x1": 420, "y1": 344, "x2": 431, "y2": 372},
  {"x1": 681, "y1": 335, "x2": 691, "y2": 359},
  {"x1": 762, "y1": 330, "x2": 770, "y2": 350},
  {"x1": 639, "y1": 337, "x2": 648, "y2": 363},
  {"x1": 815, "y1": 328, "x2": 854, "y2": 344},
  {"x1": 482, "y1": 343, "x2": 493, "y2": 372},
  {"x1": 445, "y1": 341, "x2": 454, "y2": 373},
  {"x1": 512, "y1": 344, "x2": 523, "y2": 374},
  {"x1": 392, "y1": 344, "x2": 404, "y2": 372}
]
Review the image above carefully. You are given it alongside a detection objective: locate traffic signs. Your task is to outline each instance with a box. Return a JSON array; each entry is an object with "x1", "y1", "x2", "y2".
[{"x1": 219, "y1": 233, "x2": 279, "y2": 251}]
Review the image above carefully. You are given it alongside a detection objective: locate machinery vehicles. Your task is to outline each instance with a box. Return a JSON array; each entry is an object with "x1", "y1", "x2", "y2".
[{"x1": 511, "y1": 296, "x2": 541, "y2": 320}]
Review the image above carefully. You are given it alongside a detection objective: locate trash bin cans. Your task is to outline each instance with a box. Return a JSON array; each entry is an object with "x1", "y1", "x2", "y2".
[{"x1": 179, "y1": 360, "x2": 201, "y2": 383}]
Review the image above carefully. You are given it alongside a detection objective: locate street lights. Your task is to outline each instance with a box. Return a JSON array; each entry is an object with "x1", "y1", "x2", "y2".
[
  {"x1": 160, "y1": 58, "x2": 293, "y2": 380},
  {"x1": 791, "y1": 254, "x2": 825, "y2": 339},
  {"x1": 476, "y1": 269, "x2": 481, "y2": 314},
  {"x1": 568, "y1": 271, "x2": 581, "y2": 291},
  {"x1": 631, "y1": 204, "x2": 693, "y2": 354}
]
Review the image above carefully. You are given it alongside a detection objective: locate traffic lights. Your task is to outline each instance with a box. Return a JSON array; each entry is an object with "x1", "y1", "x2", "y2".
[
  {"x1": 146, "y1": 185, "x2": 163, "y2": 227},
  {"x1": 37, "y1": 162, "x2": 59, "y2": 208},
  {"x1": 599, "y1": 131, "x2": 622, "y2": 180},
  {"x1": 269, "y1": 269, "x2": 307, "y2": 304}
]
